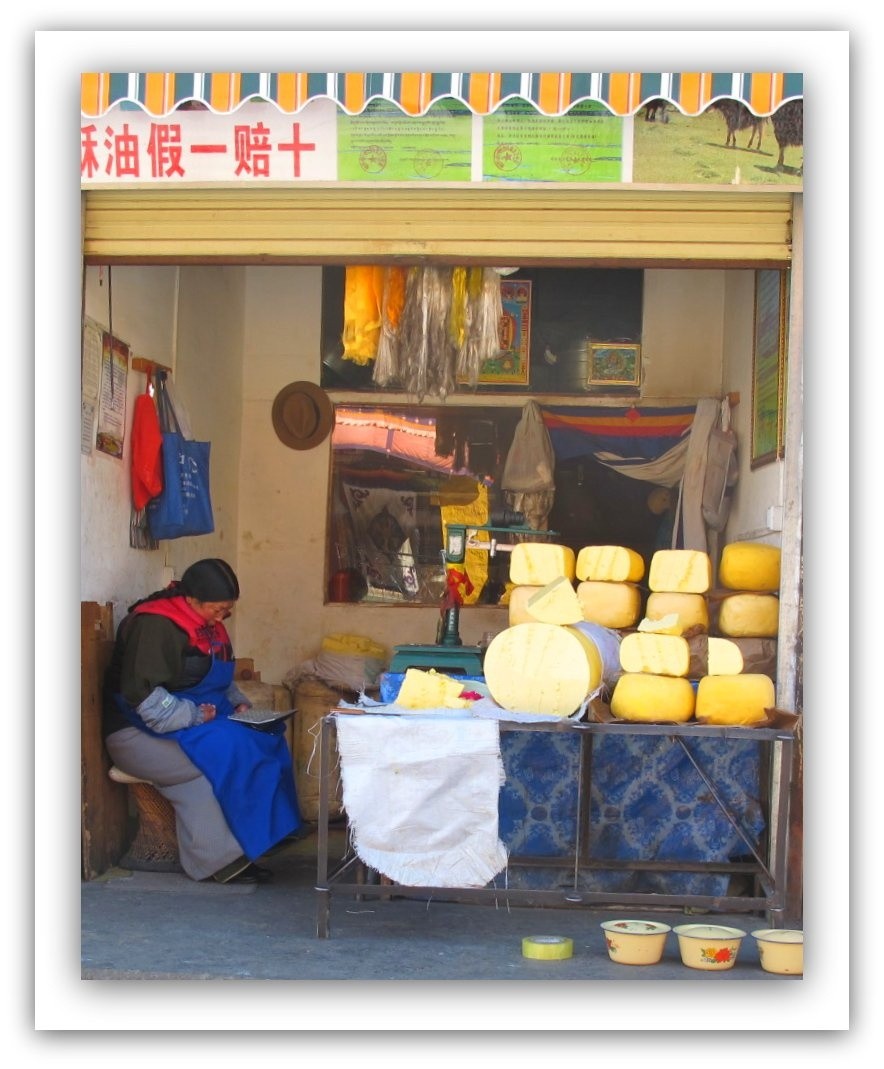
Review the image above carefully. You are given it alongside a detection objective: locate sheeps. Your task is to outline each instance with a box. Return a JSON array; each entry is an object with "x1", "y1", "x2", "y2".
[{"x1": 643, "y1": 97, "x2": 804, "y2": 175}]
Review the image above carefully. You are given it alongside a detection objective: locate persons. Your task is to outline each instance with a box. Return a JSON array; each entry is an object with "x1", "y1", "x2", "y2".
[{"x1": 102, "y1": 558, "x2": 303, "y2": 884}]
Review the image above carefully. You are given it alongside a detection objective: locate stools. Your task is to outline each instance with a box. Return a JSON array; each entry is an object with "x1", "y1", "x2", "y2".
[{"x1": 109, "y1": 766, "x2": 181, "y2": 872}]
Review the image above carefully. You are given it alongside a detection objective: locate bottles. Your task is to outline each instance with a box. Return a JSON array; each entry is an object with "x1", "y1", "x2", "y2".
[{"x1": 332, "y1": 568, "x2": 350, "y2": 602}]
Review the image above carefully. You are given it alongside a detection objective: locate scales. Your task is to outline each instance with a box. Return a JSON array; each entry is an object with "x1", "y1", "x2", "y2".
[{"x1": 387, "y1": 511, "x2": 560, "y2": 678}]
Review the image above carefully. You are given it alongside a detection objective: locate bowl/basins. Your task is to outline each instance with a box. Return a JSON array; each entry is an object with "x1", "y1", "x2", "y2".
[
  {"x1": 600, "y1": 919, "x2": 672, "y2": 964},
  {"x1": 672, "y1": 923, "x2": 747, "y2": 970},
  {"x1": 750, "y1": 928, "x2": 803, "y2": 975}
]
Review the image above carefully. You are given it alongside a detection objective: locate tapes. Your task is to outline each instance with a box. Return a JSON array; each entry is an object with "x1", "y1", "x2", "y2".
[{"x1": 522, "y1": 935, "x2": 572, "y2": 959}]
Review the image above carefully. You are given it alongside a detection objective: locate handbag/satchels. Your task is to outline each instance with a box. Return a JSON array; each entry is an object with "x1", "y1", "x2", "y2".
[
  {"x1": 147, "y1": 367, "x2": 213, "y2": 541},
  {"x1": 131, "y1": 364, "x2": 164, "y2": 512},
  {"x1": 501, "y1": 401, "x2": 558, "y2": 493}
]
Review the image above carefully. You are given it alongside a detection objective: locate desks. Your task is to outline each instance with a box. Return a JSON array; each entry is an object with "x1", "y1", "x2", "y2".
[{"x1": 316, "y1": 715, "x2": 791, "y2": 941}]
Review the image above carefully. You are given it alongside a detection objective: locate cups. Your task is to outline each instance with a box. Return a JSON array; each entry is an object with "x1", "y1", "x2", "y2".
[{"x1": 481, "y1": 632, "x2": 497, "y2": 652}]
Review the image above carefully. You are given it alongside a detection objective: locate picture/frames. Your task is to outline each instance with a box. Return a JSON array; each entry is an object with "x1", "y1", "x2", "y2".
[
  {"x1": 454, "y1": 278, "x2": 532, "y2": 385},
  {"x1": 586, "y1": 343, "x2": 640, "y2": 386}
]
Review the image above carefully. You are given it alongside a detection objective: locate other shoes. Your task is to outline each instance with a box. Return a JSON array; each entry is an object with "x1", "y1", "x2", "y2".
[{"x1": 230, "y1": 863, "x2": 274, "y2": 883}]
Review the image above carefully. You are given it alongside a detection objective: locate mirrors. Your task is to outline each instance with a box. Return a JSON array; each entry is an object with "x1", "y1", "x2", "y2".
[{"x1": 323, "y1": 401, "x2": 696, "y2": 609}]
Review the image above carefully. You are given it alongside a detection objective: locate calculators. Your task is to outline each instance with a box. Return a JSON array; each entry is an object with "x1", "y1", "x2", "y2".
[{"x1": 229, "y1": 707, "x2": 299, "y2": 729}]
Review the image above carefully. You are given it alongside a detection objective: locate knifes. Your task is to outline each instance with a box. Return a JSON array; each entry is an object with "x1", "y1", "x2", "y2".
[{"x1": 330, "y1": 708, "x2": 403, "y2": 716}]
